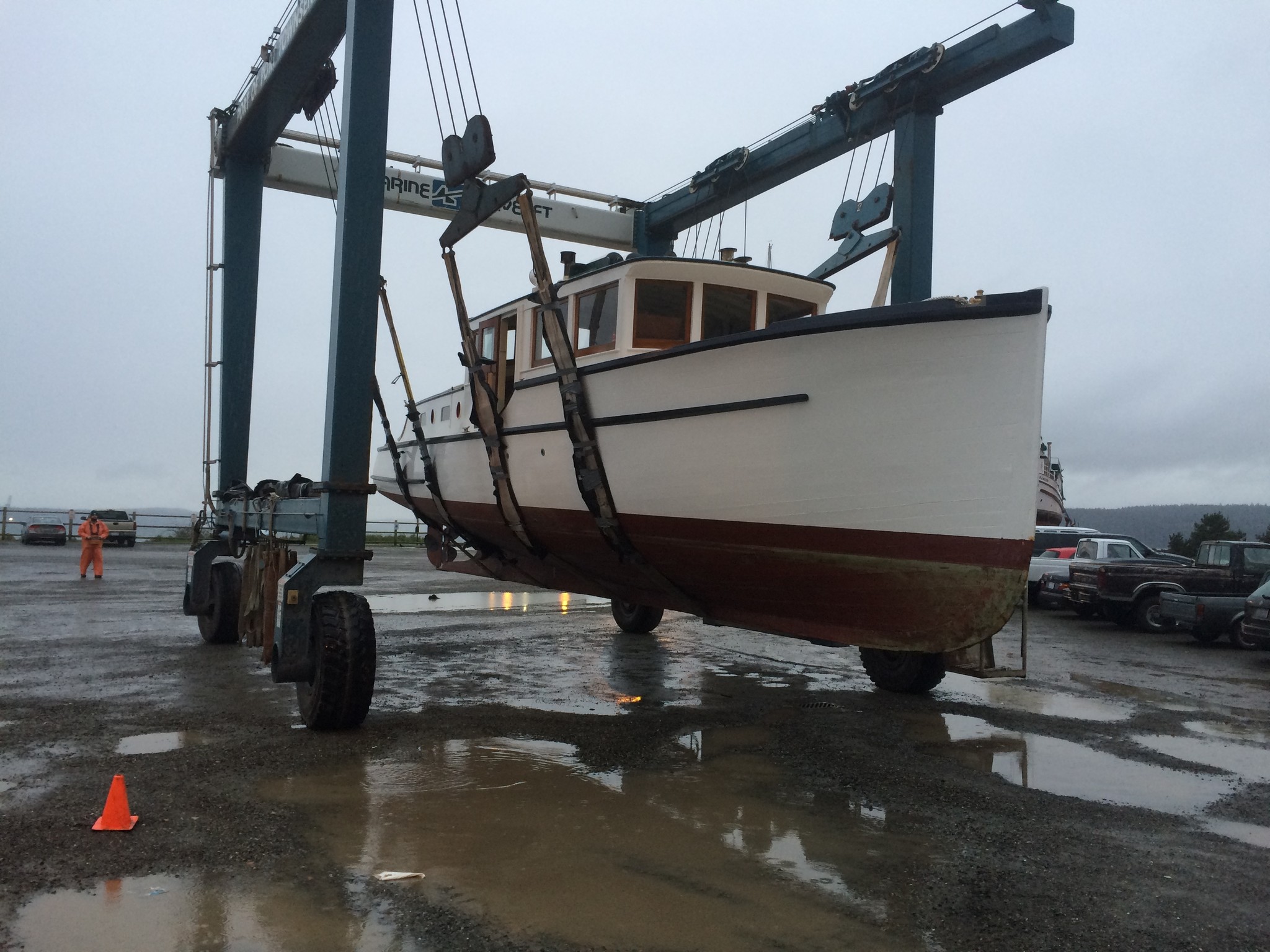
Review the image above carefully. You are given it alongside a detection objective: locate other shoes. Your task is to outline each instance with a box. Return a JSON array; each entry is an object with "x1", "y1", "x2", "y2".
[
  {"x1": 81, "y1": 574, "x2": 86, "y2": 577},
  {"x1": 94, "y1": 575, "x2": 103, "y2": 579}
]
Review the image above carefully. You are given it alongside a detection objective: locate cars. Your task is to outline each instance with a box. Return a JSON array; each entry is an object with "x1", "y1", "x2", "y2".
[{"x1": 20, "y1": 516, "x2": 66, "y2": 545}]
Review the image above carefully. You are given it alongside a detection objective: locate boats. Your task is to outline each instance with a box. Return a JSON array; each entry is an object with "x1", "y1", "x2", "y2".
[
  {"x1": 370, "y1": 248, "x2": 1051, "y2": 651},
  {"x1": 1036, "y1": 436, "x2": 1066, "y2": 527}
]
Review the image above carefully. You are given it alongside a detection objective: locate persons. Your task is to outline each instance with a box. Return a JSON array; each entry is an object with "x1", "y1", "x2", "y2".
[{"x1": 78, "y1": 510, "x2": 109, "y2": 578}]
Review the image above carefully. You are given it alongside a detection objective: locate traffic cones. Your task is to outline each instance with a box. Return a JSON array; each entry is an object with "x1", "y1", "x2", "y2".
[{"x1": 91, "y1": 774, "x2": 139, "y2": 831}]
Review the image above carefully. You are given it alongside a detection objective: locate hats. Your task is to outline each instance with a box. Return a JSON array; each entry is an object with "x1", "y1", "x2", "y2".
[{"x1": 90, "y1": 510, "x2": 97, "y2": 515}]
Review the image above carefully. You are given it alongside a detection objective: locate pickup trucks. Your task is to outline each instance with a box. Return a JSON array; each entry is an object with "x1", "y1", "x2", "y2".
[
  {"x1": 1022, "y1": 525, "x2": 1270, "y2": 651},
  {"x1": 81, "y1": 508, "x2": 137, "y2": 547}
]
